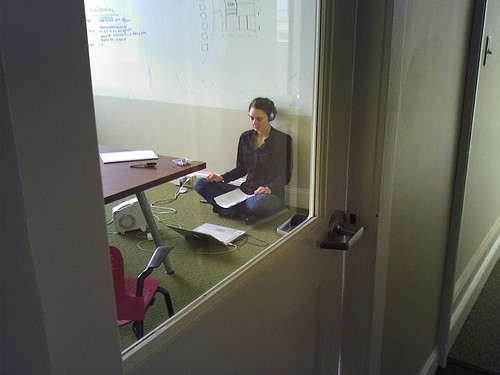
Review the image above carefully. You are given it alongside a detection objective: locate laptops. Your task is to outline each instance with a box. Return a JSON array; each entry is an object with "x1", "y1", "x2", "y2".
[{"x1": 167, "y1": 222, "x2": 247, "y2": 246}]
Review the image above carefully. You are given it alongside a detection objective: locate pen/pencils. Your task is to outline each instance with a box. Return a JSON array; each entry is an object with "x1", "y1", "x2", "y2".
[{"x1": 129, "y1": 162, "x2": 157, "y2": 168}]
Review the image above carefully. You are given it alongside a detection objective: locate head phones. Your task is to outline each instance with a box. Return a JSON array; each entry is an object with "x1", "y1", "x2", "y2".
[{"x1": 248, "y1": 103, "x2": 275, "y2": 121}]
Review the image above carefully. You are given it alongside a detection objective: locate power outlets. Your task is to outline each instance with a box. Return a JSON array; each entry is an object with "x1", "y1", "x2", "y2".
[{"x1": 182, "y1": 175, "x2": 195, "y2": 188}]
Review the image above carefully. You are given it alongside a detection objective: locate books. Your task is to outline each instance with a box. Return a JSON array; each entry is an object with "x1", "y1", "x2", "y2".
[{"x1": 100, "y1": 149, "x2": 159, "y2": 164}]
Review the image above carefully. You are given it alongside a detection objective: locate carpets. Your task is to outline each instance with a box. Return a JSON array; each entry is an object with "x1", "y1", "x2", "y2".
[{"x1": 103, "y1": 181, "x2": 309, "y2": 353}]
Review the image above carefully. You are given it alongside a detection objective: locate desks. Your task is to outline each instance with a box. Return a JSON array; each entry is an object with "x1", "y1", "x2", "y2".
[{"x1": 99, "y1": 144, "x2": 207, "y2": 273}]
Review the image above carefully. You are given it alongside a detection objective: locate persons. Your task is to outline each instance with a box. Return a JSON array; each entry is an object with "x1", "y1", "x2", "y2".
[{"x1": 192, "y1": 97, "x2": 294, "y2": 224}]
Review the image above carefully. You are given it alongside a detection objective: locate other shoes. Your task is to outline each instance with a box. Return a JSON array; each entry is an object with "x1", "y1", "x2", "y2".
[
  {"x1": 212, "y1": 206, "x2": 218, "y2": 213},
  {"x1": 244, "y1": 214, "x2": 257, "y2": 225}
]
